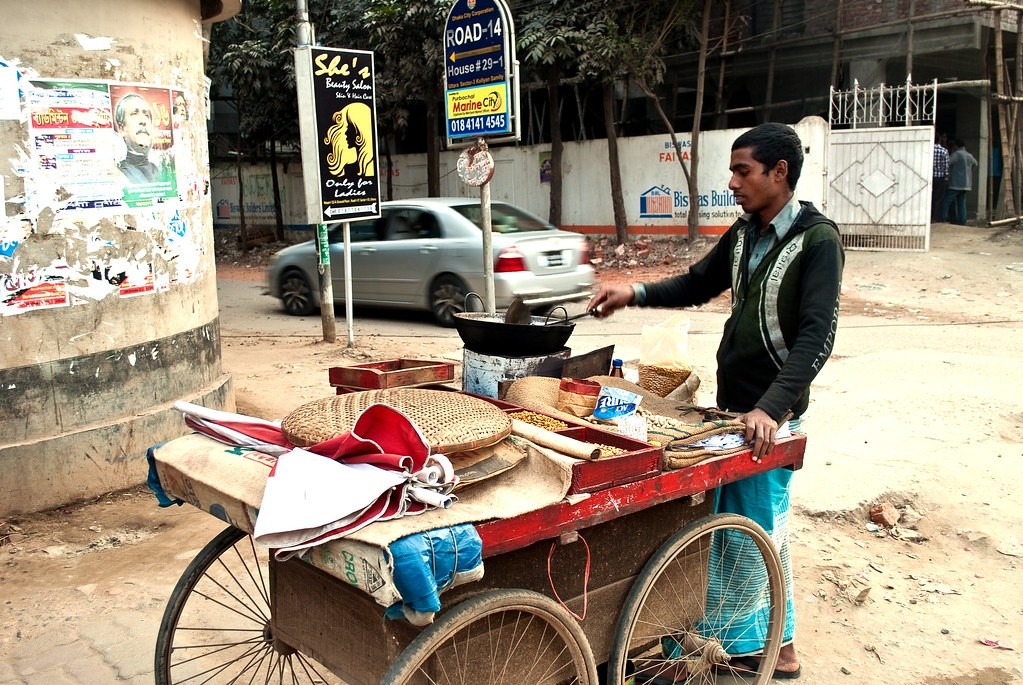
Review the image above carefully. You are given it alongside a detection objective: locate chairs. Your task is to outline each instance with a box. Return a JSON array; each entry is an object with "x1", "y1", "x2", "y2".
[{"x1": 392, "y1": 215, "x2": 418, "y2": 241}]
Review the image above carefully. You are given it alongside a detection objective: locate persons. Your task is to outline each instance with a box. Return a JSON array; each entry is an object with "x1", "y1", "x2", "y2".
[
  {"x1": 932, "y1": 133, "x2": 977, "y2": 226},
  {"x1": 112, "y1": 93, "x2": 187, "y2": 184},
  {"x1": 587, "y1": 122, "x2": 846, "y2": 679}
]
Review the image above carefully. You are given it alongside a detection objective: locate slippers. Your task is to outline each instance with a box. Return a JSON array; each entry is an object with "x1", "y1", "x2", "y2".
[
  {"x1": 711, "y1": 653, "x2": 801, "y2": 678},
  {"x1": 633, "y1": 652, "x2": 693, "y2": 685}
]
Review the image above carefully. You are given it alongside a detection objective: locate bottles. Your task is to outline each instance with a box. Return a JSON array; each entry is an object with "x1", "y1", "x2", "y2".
[{"x1": 611, "y1": 359, "x2": 624, "y2": 379}]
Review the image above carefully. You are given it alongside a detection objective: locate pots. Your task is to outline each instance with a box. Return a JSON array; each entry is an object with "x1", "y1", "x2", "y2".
[{"x1": 453, "y1": 292, "x2": 576, "y2": 357}]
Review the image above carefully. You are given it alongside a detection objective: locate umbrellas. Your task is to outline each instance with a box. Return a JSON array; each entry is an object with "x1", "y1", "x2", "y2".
[{"x1": 170, "y1": 400, "x2": 459, "y2": 561}]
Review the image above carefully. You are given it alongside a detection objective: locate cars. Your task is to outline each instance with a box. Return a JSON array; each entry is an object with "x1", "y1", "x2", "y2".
[{"x1": 265, "y1": 196, "x2": 603, "y2": 330}]
